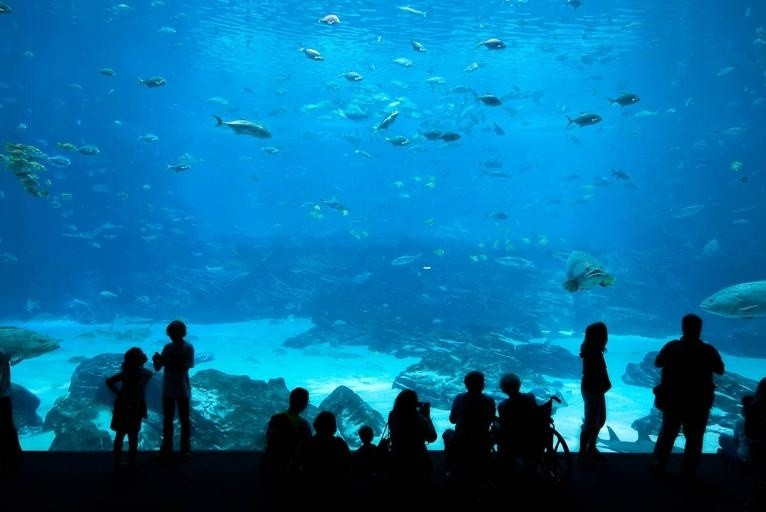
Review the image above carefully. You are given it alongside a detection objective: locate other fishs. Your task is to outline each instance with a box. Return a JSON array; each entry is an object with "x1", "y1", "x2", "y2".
[{"x1": 0, "y1": 0, "x2": 766, "y2": 366}]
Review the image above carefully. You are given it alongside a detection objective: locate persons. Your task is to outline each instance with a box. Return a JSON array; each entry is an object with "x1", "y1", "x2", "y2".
[
  {"x1": 267, "y1": 370, "x2": 547, "y2": 504},
  {"x1": 651, "y1": 314, "x2": 724, "y2": 470},
  {"x1": 105, "y1": 348, "x2": 152, "y2": 460},
  {"x1": 0, "y1": 352, "x2": 24, "y2": 456},
  {"x1": 742, "y1": 378, "x2": 766, "y2": 470},
  {"x1": 575, "y1": 322, "x2": 609, "y2": 464},
  {"x1": 153, "y1": 320, "x2": 194, "y2": 458}
]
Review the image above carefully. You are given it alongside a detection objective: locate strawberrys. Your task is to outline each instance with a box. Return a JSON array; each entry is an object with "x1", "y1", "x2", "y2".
[{"x1": 487, "y1": 395, "x2": 574, "y2": 486}]
